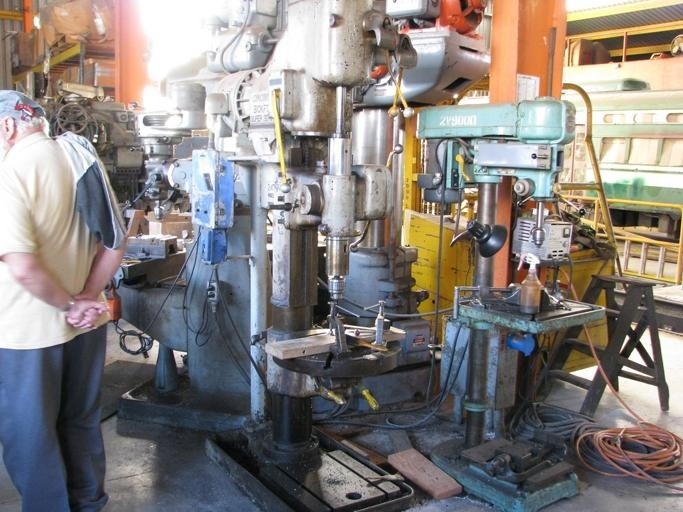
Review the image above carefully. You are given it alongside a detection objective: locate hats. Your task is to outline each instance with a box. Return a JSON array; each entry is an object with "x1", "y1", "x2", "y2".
[{"x1": 0, "y1": 89, "x2": 46, "y2": 123}]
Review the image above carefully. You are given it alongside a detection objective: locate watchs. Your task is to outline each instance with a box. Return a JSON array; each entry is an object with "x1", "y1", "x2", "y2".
[{"x1": 61, "y1": 295, "x2": 76, "y2": 311}]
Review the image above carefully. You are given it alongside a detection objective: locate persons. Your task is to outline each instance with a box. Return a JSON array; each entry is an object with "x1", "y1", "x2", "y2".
[{"x1": 1, "y1": 91, "x2": 125, "y2": 512}]
